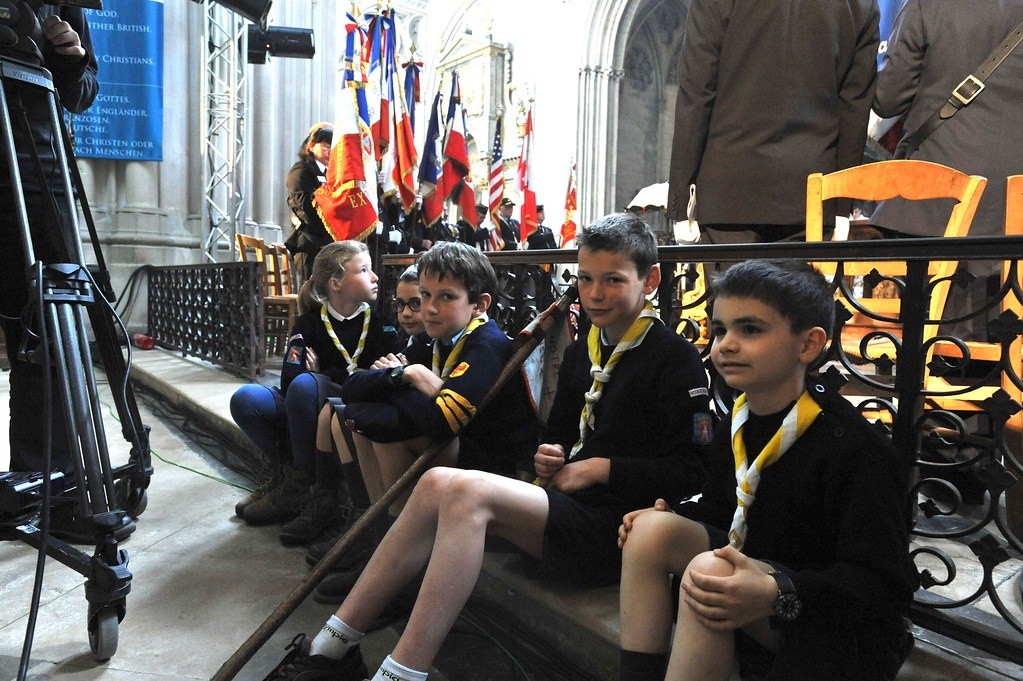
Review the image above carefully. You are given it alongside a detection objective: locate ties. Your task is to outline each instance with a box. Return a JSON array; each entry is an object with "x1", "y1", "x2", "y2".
[
  {"x1": 568, "y1": 298, "x2": 657, "y2": 460},
  {"x1": 432, "y1": 310, "x2": 489, "y2": 382},
  {"x1": 320, "y1": 301, "x2": 370, "y2": 376},
  {"x1": 725, "y1": 389, "x2": 825, "y2": 552}
]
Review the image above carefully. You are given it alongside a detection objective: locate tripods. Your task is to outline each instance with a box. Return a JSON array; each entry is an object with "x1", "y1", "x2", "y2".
[{"x1": 0, "y1": 0, "x2": 155, "y2": 662}]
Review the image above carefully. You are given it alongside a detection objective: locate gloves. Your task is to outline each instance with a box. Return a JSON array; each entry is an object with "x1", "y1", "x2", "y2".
[
  {"x1": 832, "y1": 215, "x2": 850, "y2": 242},
  {"x1": 672, "y1": 218, "x2": 701, "y2": 244}
]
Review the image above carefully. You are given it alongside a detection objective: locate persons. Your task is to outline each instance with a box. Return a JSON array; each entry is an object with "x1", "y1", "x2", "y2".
[
  {"x1": 614, "y1": 260, "x2": 912, "y2": 681},
  {"x1": 869, "y1": 0, "x2": 1023, "y2": 507},
  {"x1": 852, "y1": 204, "x2": 869, "y2": 220},
  {"x1": 261, "y1": 213, "x2": 709, "y2": 681},
  {"x1": 0, "y1": 0, "x2": 100, "y2": 500},
  {"x1": 285, "y1": 121, "x2": 334, "y2": 291},
  {"x1": 376, "y1": 171, "x2": 557, "y2": 255},
  {"x1": 668, "y1": 0, "x2": 878, "y2": 306},
  {"x1": 229, "y1": 241, "x2": 538, "y2": 635}
]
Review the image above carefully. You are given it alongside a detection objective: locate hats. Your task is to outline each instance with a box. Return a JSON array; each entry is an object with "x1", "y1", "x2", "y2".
[
  {"x1": 314, "y1": 129, "x2": 332, "y2": 144},
  {"x1": 475, "y1": 204, "x2": 488, "y2": 216},
  {"x1": 536, "y1": 205, "x2": 543, "y2": 212},
  {"x1": 502, "y1": 198, "x2": 515, "y2": 206}
]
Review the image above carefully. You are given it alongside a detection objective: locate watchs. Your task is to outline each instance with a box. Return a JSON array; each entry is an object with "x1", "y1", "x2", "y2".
[
  {"x1": 768, "y1": 570, "x2": 802, "y2": 623},
  {"x1": 390, "y1": 365, "x2": 407, "y2": 385}
]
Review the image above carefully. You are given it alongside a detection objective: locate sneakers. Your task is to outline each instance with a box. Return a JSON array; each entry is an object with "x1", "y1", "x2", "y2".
[
  {"x1": 279, "y1": 476, "x2": 356, "y2": 546},
  {"x1": 305, "y1": 511, "x2": 391, "y2": 570},
  {"x1": 236, "y1": 458, "x2": 320, "y2": 528},
  {"x1": 264, "y1": 633, "x2": 370, "y2": 681}
]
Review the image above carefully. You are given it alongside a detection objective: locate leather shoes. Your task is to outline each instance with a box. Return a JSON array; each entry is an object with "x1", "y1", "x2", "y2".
[{"x1": 37, "y1": 501, "x2": 136, "y2": 544}]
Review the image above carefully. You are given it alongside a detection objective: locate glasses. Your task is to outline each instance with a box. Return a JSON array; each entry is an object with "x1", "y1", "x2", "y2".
[{"x1": 388, "y1": 297, "x2": 421, "y2": 313}]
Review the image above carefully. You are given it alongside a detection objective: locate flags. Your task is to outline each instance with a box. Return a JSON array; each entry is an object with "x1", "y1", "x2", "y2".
[
  {"x1": 488, "y1": 116, "x2": 505, "y2": 251},
  {"x1": 560, "y1": 173, "x2": 578, "y2": 248},
  {"x1": 517, "y1": 109, "x2": 539, "y2": 246},
  {"x1": 313, "y1": 8, "x2": 476, "y2": 243}
]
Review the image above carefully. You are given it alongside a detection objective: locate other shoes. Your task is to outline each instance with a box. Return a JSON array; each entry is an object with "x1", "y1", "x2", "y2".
[{"x1": 311, "y1": 546, "x2": 423, "y2": 633}]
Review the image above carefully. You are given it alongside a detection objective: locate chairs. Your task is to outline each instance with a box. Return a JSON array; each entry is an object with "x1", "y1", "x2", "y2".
[
  {"x1": 236, "y1": 234, "x2": 299, "y2": 358},
  {"x1": 803, "y1": 158, "x2": 987, "y2": 424},
  {"x1": 934, "y1": 176, "x2": 1023, "y2": 430}
]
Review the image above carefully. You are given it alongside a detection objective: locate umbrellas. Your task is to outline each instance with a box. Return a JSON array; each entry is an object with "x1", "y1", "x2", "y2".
[{"x1": 624, "y1": 180, "x2": 670, "y2": 213}]
